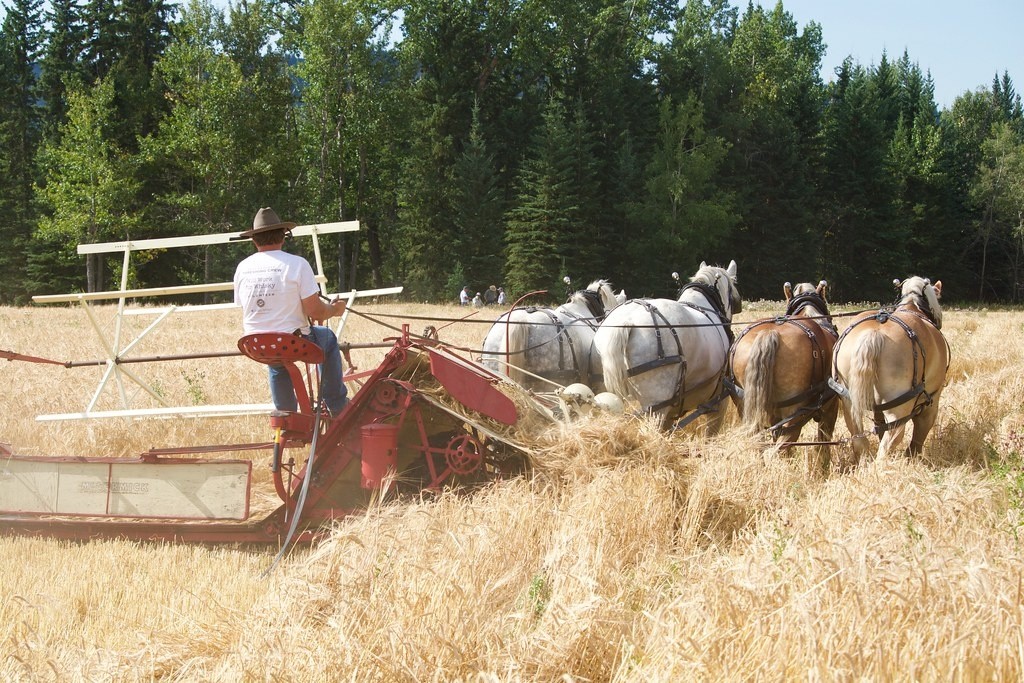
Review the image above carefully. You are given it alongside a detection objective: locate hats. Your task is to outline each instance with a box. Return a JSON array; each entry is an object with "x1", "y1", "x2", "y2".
[
  {"x1": 490, "y1": 285, "x2": 496, "y2": 291},
  {"x1": 497, "y1": 287, "x2": 503, "y2": 291},
  {"x1": 476, "y1": 293, "x2": 482, "y2": 296},
  {"x1": 239, "y1": 207, "x2": 297, "y2": 236}
]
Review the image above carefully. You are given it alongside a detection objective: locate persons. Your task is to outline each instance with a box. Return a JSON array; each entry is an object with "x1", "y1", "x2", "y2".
[
  {"x1": 459, "y1": 286, "x2": 470, "y2": 305},
  {"x1": 472, "y1": 291, "x2": 484, "y2": 306},
  {"x1": 497, "y1": 287, "x2": 506, "y2": 305},
  {"x1": 234, "y1": 205, "x2": 350, "y2": 416},
  {"x1": 484, "y1": 285, "x2": 497, "y2": 304}
]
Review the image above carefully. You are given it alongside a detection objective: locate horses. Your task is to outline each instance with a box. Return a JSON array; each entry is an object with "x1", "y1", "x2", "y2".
[{"x1": 479, "y1": 259, "x2": 950, "y2": 478}]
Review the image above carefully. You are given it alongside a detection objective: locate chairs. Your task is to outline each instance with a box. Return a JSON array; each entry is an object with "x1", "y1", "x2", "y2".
[{"x1": 237, "y1": 334, "x2": 325, "y2": 416}]
